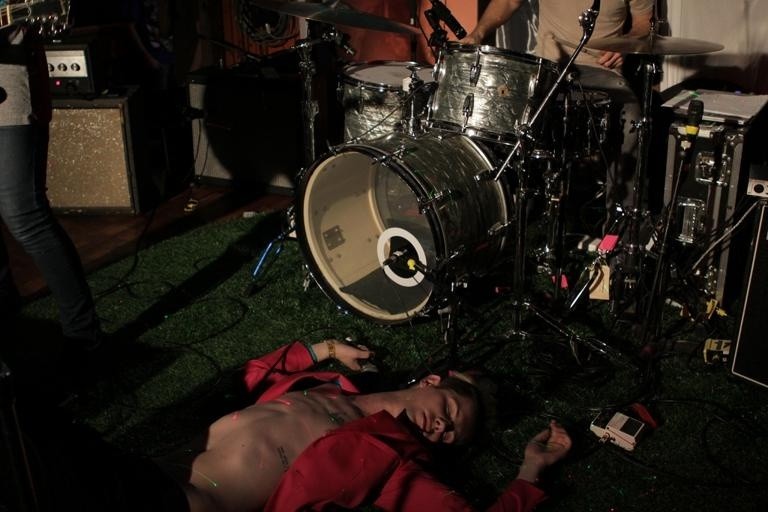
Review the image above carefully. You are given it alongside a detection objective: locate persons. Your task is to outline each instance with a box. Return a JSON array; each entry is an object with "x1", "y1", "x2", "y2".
[
  {"x1": 53, "y1": 336, "x2": 573, "y2": 510},
  {"x1": 457, "y1": 0, "x2": 654, "y2": 275},
  {"x1": 0, "y1": 1, "x2": 102, "y2": 362}
]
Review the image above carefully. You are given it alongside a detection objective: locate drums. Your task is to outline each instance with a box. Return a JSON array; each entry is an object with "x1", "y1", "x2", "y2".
[
  {"x1": 343, "y1": 60, "x2": 439, "y2": 146},
  {"x1": 526, "y1": 84, "x2": 626, "y2": 162},
  {"x1": 293, "y1": 126, "x2": 514, "y2": 325},
  {"x1": 425, "y1": 40, "x2": 558, "y2": 148}
]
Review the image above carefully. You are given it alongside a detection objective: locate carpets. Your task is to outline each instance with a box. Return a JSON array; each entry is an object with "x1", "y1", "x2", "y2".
[{"x1": 0, "y1": 209, "x2": 768, "y2": 512}]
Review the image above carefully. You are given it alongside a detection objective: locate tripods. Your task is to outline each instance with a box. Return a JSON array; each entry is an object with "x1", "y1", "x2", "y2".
[
  {"x1": 242, "y1": 29, "x2": 357, "y2": 300},
  {"x1": 401, "y1": 121, "x2": 638, "y2": 390},
  {"x1": 525, "y1": 63, "x2": 739, "y2": 366}
]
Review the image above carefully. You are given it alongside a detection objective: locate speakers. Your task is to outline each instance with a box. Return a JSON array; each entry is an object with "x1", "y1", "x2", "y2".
[{"x1": 38, "y1": 79, "x2": 168, "y2": 216}]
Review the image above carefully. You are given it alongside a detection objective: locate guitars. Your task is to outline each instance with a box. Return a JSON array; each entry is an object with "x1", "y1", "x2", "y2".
[{"x1": 0, "y1": 0, "x2": 71, "y2": 47}]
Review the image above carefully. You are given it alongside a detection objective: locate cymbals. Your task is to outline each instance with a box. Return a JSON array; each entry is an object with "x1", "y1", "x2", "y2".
[
  {"x1": 584, "y1": 34, "x2": 724, "y2": 56},
  {"x1": 267, "y1": 0, "x2": 422, "y2": 35}
]
